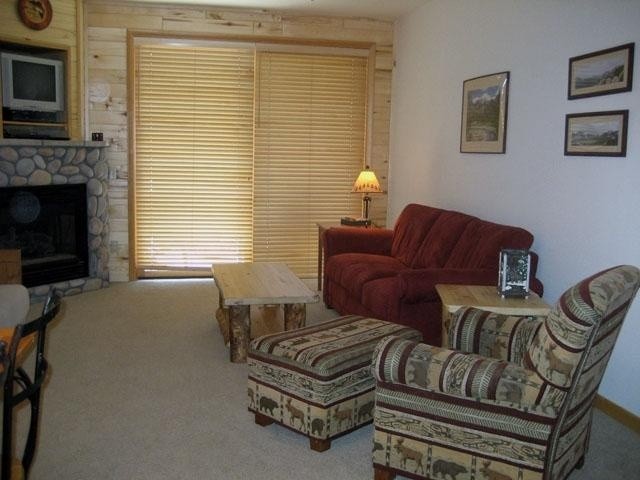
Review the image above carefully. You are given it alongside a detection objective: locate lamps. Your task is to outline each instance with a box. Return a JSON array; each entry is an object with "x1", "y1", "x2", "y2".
[{"x1": 349, "y1": 164, "x2": 384, "y2": 221}]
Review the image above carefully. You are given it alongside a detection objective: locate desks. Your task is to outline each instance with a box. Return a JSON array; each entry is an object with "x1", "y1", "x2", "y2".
[
  {"x1": 431, "y1": 281, "x2": 553, "y2": 352},
  {"x1": 314, "y1": 216, "x2": 382, "y2": 293}
]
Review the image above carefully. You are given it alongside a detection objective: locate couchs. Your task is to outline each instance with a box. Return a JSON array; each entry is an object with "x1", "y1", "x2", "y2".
[{"x1": 316, "y1": 199, "x2": 545, "y2": 357}]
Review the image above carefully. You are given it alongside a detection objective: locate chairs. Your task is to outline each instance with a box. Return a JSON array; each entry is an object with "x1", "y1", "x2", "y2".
[
  {"x1": 369, "y1": 264, "x2": 640, "y2": 480},
  {"x1": 0, "y1": 282, "x2": 65, "y2": 480}
]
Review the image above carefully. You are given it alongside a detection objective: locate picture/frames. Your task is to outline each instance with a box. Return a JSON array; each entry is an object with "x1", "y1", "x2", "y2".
[
  {"x1": 564, "y1": 41, "x2": 634, "y2": 160},
  {"x1": 457, "y1": 68, "x2": 512, "y2": 155}
]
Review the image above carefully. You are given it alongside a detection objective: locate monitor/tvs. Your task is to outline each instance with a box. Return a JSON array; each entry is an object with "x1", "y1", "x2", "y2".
[{"x1": 0, "y1": 52, "x2": 65, "y2": 115}]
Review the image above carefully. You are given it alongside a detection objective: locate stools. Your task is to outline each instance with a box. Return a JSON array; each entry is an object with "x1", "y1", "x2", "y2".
[{"x1": 243, "y1": 310, "x2": 426, "y2": 455}]
